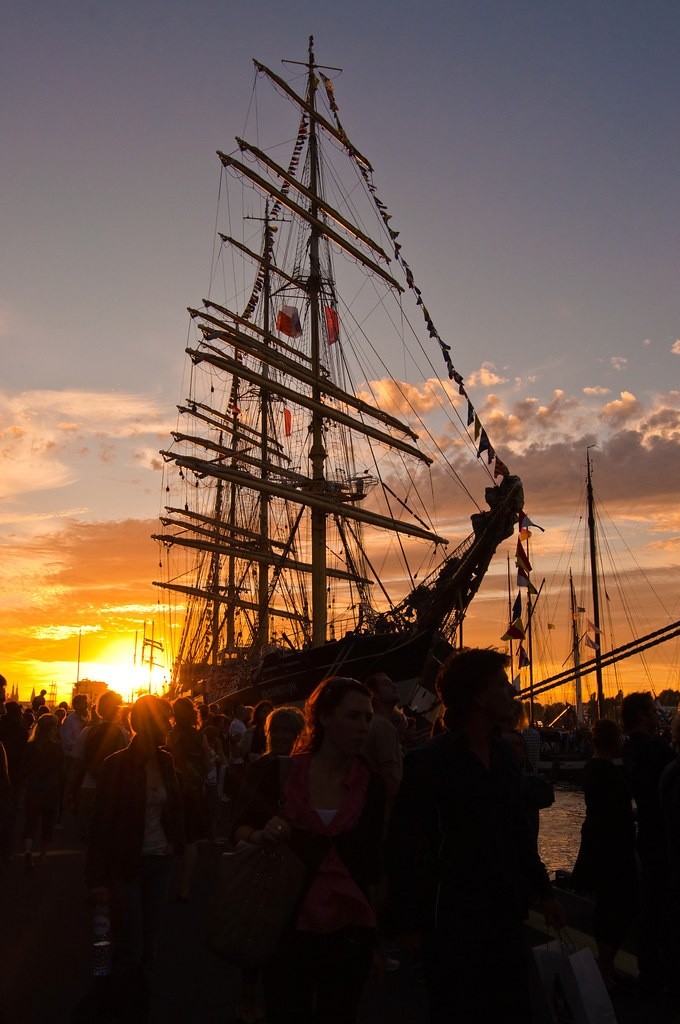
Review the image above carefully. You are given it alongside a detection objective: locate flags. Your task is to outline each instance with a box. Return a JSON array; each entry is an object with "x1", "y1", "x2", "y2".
[
  {"x1": 284, "y1": 408, "x2": 291, "y2": 436},
  {"x1": 276, "y1": 305, "x2": 303, "y2": 339},
  {"x1": 325, "y1": 306, "x2": 339, "y2": 345}
]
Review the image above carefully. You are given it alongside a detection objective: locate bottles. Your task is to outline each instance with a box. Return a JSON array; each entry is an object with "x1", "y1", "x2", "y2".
[{"x1": 91, "y1": 902, "x2": 111, "y2": 976}]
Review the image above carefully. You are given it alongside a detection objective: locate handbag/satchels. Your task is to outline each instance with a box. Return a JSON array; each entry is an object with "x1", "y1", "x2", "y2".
[
  {"x1": 213, "y1": 746, "x2": 228, "y2": 765},
  {"x1": 532, "y1": 919, "x2": 617, "y2": 1024},
  {"x1": 210, "y1": 840, "x2": 307, "y2": 966}
]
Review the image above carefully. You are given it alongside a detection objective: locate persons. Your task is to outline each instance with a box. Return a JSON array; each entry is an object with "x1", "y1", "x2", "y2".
[{"x1": 0, "y1": 648, "x2": 680, "y2": 1024}]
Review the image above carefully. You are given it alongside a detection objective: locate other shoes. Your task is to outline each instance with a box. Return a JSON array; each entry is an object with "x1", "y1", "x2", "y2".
[
  {"x1": 178, "y1": 881, "x2": 191, "y2": 903},
  {"x1": 216, "y1": 795, "x2": 229, "y2": 801},
  {"x1": 24, "y1": 851, "x2": 34, "y2": 872}
]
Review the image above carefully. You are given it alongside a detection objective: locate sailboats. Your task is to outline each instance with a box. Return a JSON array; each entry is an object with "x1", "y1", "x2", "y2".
[{"x1": 152, "y1": 34, "x2": 517, "y2": 737}]
